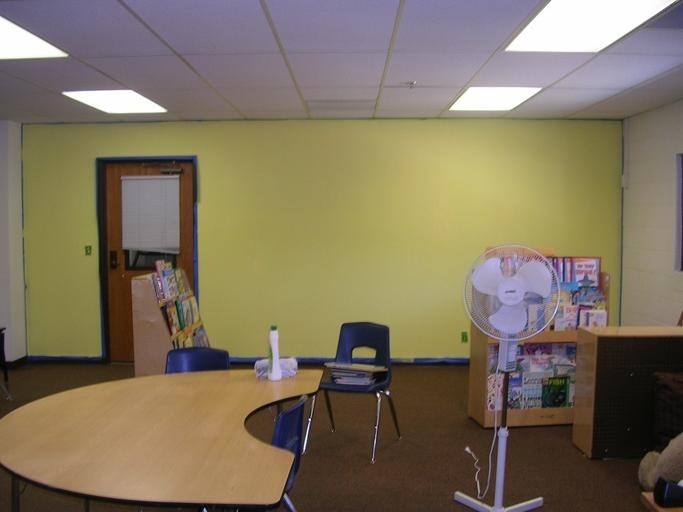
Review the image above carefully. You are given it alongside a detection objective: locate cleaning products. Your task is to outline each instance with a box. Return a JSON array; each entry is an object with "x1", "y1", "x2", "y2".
[{"x1": 266, "y1": 325, "x2": 282, "y2": 382}]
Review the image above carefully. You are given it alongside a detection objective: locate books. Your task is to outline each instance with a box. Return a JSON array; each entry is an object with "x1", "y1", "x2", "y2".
[
  {"x1": 152, "y1": 260, "x2": 210, "y2": 349},
  {"x1": 488, "y1": 255, "x2": 607, "y2": 411},
  {"x1": 324, "y1": 362, "x2": 389, "y2": 385}
]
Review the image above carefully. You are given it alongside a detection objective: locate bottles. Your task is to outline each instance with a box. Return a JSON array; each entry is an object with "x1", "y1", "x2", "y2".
[{"x1": 268, "y1": 325, "x2": 282, "y2": 380}]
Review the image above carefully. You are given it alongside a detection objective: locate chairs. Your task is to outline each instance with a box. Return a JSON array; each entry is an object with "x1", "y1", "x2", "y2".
[
  {"x1": 202, "y1": 395, "x2": 310, "y2": 512},
  {"x1": 164, "y1": 346, "x2": 228, "y2": 374},
  {"x1": 641, "y1": 489, "x2": 682, "y2": 512},
  {"x1": 300, "y1": 322, "x2": 402, "y2": 464}
]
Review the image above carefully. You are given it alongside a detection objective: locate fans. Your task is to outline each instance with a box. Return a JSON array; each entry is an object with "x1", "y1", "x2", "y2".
[{"x1": 453, "y1": 242, "x2": 562, "y2": 512}]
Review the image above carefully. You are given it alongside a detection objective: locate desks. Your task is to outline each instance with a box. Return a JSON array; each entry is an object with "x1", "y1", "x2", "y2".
[{"x1": 0, "y1": 365, "x2": 325, "y2": 512}]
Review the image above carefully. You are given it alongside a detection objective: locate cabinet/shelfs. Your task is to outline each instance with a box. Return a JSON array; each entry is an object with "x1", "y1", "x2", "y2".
[
  {"x1": 571, "y1": 326, "x2": 682, "y2": 458},
  {"x1": 470, "y1": 256, "x2": 611, "y2": 429},
  {"x1": 130, "y1": 274, "x2": 174, "y2": 376}
]
